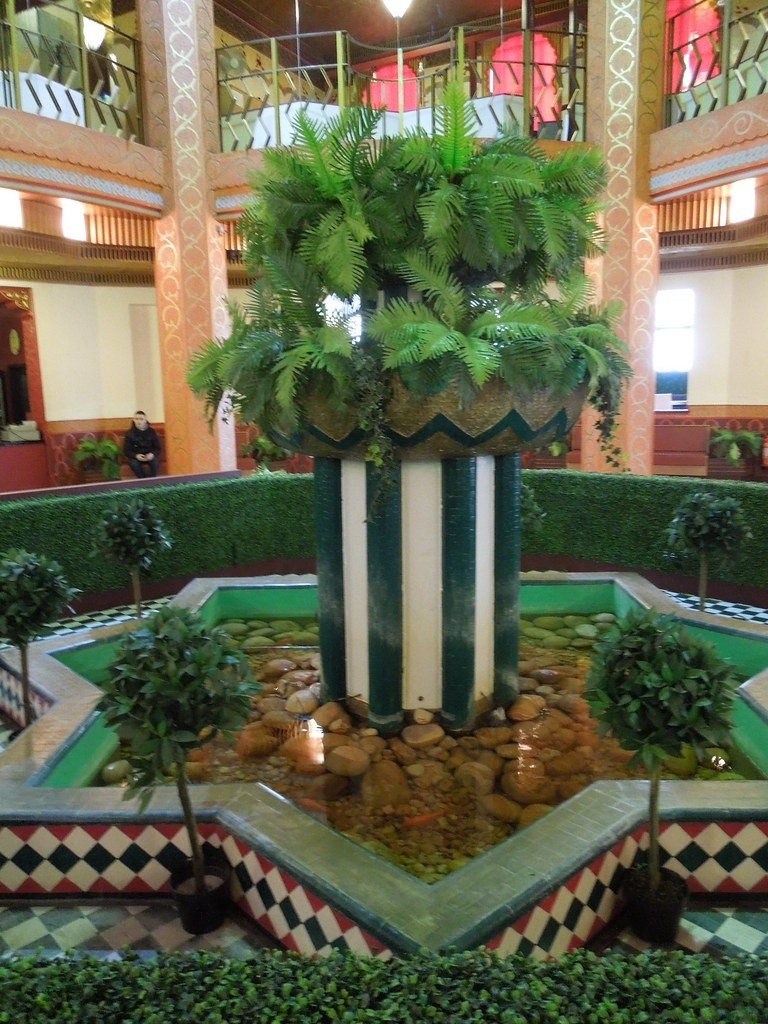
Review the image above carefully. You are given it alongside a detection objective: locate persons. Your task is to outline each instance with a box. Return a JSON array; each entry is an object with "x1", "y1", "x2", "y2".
[{"x1": 123, "y1": 411, "x2": 162, "y2": 478}]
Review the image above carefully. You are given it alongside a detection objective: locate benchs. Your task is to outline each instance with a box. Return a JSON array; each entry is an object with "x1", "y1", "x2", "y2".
[
  {"x1": 118, "y1": 428, "x2": 168, "y2": 481},
  {"x1": 651, "y1": 424, "x2": 710, "y2": 477}
]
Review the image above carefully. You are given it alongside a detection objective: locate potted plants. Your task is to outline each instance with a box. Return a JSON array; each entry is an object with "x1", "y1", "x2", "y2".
[
  {"x1": 240, "y1": 433, "x2": 295, "y2": 476},
  {"x1": 528, "y1": 439, "x2": 570, "y2": 471},
  {"x1": 707, "y1": 427, "x2": 763, "y2": 481},
  {"x1": 73, "y1": 435, "x2": 124, "y2": 486},
  {"x1": 182, "y1": 64, "x2": 635, "y2": 740},
  {"x1": 581, "y1": 604, "x2": 741, "y2": 945},
  {"x1": 662, "y1": 493, "x2": 754, "y2": 612},
  {"x1": 94, "y1": 606, "x2": 263, "y2": 935},
  {"x1": 0, "y1": 545, "x2": 84, "y2": 743},
  {"x1": 99, "y1": 499, "x2": 175, "y2": 617}
]
